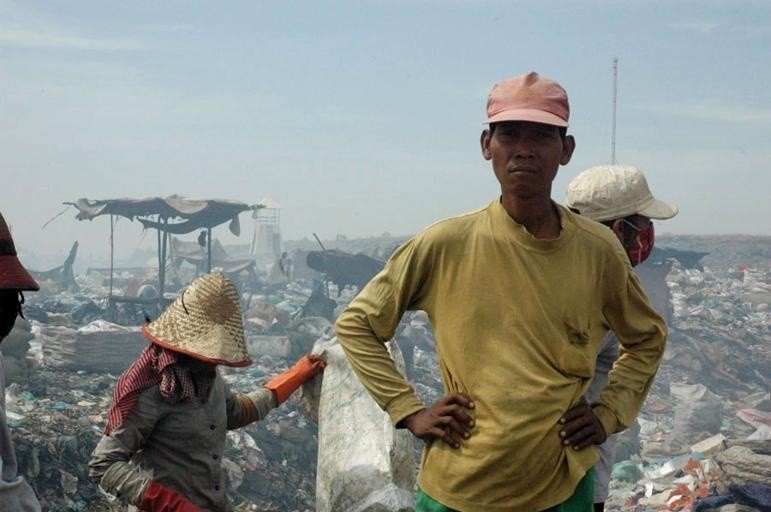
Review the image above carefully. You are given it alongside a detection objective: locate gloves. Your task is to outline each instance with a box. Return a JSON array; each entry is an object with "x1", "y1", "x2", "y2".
[
  {"x1": 137, "y1": 478, "x2": 206, "y2": 510},
  {"x1": 264, "y1": 352, "x2": 328, "y2": 408}
]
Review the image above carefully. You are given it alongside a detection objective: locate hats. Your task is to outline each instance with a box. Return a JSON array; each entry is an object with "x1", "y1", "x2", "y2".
[
  {"x1": 139, "y1": 267, "x2": 253, "y2": 371},
  {"x1": 562, "y1": 161, "x2": 682, "y2": 220},
  {"x1": 480, "y1": 68, "x2": 572, "y2": 134},
  {"x1": 0, "y1": 210, "x2": 43, "y2": 294}
]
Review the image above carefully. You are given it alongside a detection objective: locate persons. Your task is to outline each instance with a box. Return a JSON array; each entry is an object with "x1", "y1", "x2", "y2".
[
  {"x1": 1, "y1": 211, "x2": 50, "y2": 512},
  {"x1": 82, "y1": 272, "x2": 330, "y2": 510},
  {"x1": 562, "y1": 162, "x2": 680, "y2": 510},
  {"x1": 331, "y1": 69, "x2": 670, "y2": 510}
]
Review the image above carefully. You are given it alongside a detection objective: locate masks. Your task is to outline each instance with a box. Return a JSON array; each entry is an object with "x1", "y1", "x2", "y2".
[{"x1": 625, "y1": 220, "x2": 657, "y2": 268}]
